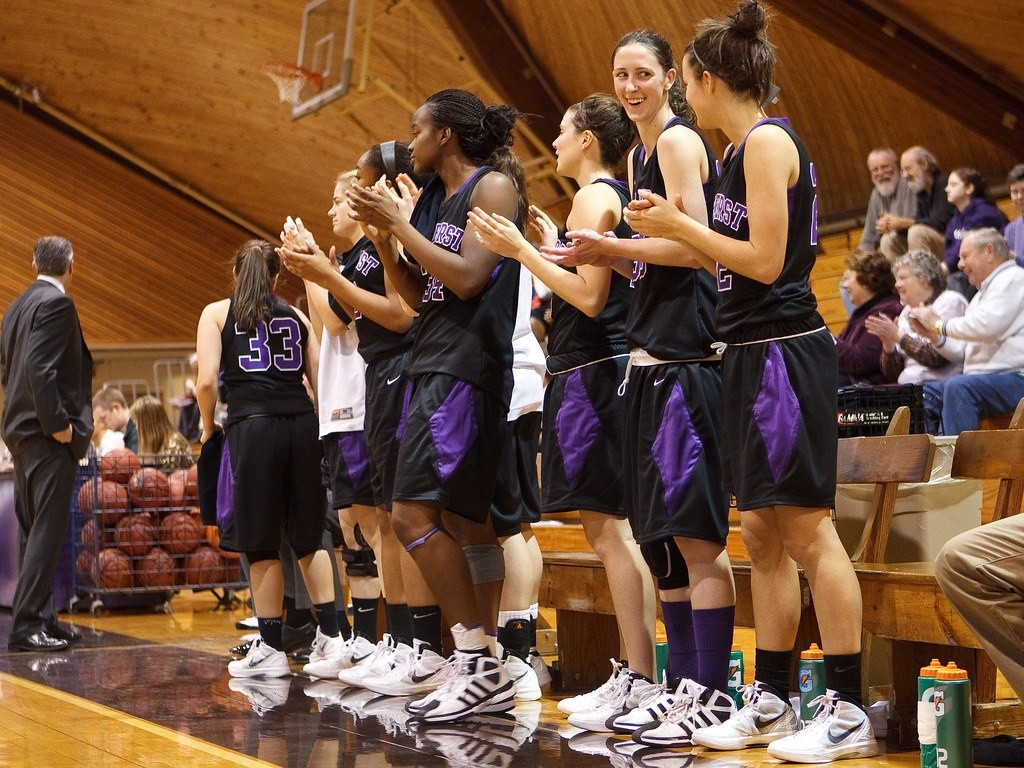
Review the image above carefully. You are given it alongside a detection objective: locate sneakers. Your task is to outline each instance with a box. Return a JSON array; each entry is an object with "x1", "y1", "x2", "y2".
[
  {"x1": 338, "y1": 634, "x2": 450, "y2": 696},
  {"x1": 496, "y1": 642, "x2": 552, "y2": 701},
  {"x1": 405, "y1": 649, "x2": 517, "y2": 722},
  {"x1": 766, "y1": 689, "x2": 879, "y2": 763},
  {"x1": 691, "y1": 680, "x2": 799, "y2": 751},
  {"x1": 557, "y1": 658, "x2": 651, "y2": 732},
  {"x1": 303, "y1": 626, "x2": 377, "y2": 678},
  {"x1": 228, "y1": 637, "x2": 292, "y2": 678},
  {"x1": 605, "y1": 669, "x2": 737, "y2": 748}
]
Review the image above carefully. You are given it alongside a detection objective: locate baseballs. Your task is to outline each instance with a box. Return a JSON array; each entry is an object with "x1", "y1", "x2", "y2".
[{"x1": 129, "y1": 467, "x2": 169, "y2": 510}]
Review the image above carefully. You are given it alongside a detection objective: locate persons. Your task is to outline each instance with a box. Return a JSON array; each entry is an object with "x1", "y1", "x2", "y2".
[
  {"x1": 467, "y1": 94, "x2": 657, "y2": 732},
  {"x1": 0, "y1": 236, "x2": 95, "y2": 653},
  {"x1": 92, "y1": 387, "x2": 138, "y2": 455},
  {"x1": 195, "y1": 240, "x2": 343, "y2": 678},
  {"x1": 834, "y1": 146, "x2": 1024, "y2": 436},
  {"x1": 92, "y1": 390, "x2": 126, "y2": 457},
  {"x1": 623, "y1": 0, "x2": 879, "y2": 763},
  {"x1": 129, "y1": 395, "x2": 195, "y2": 469},
  {"x1": 539, "y1": 28, "x2": 738, "y2": 747},
  {"x1": 274, "y1": 139, "x2": 453, "y2": 696},
  {"x1": 345, "y1": 88, "x2": 532, "y2": 724},
  {"x1": 280, "y1": 167, "x2": 386, "y2": 679},
  {"x1": 483, "y1": 146, "x2": 551, "y2": 700},
  {"x1": 933, "y1": 512, "x2": 1024, "y2": 706}
]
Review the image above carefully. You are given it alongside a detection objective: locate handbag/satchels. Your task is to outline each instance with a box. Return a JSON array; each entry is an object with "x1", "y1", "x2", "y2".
[{"x1": 837, "y1": 384, "x2": 926, "y2": 439}]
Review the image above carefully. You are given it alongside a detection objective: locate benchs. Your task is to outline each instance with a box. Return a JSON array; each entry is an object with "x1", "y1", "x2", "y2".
[{"x1": 377, "y1": 397, "x2": 1024, "y2": 741}]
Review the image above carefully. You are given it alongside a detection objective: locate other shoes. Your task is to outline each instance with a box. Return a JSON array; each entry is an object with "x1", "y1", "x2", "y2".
[
  {"x1": 235, "y1": 616, "x2": 260, "y2": 630},
  {"x1": 283, "y1": 620, "x2": 314, "y2": 665}
]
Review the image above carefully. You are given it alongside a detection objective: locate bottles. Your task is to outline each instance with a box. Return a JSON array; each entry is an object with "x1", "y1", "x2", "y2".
[
  {"x1": 799, "y1": 642, "x2": 824, "y2": 722},
  {"x1": 917, "y1": 658, "x2": 944, "y2": 768},
  {"x1": 655, "y1": 634, "x2": 668, "y2": 684},
  {"x1": 933, "y1": 661, "x2": 972, "y2": 768},
  {"x1": 728, "y1": 644, "x2": 744, "y2": 709}
]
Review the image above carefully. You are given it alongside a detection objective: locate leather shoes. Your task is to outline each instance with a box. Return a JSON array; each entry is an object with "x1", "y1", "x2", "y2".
[
  {"x1": 8, "y1": 632, "x2": 69, "y2": 651},
  {"x1": 43, "y1": 626, "x2": 84, "y2": 640}
]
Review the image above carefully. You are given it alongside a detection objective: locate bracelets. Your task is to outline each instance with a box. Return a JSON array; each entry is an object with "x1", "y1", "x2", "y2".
[{"x1": 934, "y1": 319, "x2": 944, "y2": 335}]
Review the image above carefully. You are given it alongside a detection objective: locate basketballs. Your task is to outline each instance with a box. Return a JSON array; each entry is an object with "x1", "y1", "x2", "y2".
[
  {"x1": 90, "y1": 548, "x2": 132, "y2": 587},
  {"x1": 115, "y1": 516, "x2": 158, "y2": 561},
  {"x1": 81, "y1": 519, "x2": 115, "y2": 555},
  {"x1": 166, "y1": 470, "x2": 188, "y2": 506},
  {"x1": 159, "y1": 512, "x2": 201, "y2": 557},
  {"x1": 228, "y1": 561, "x2": 248, "y2": 589},
  {"x1": 101, "y1": 447, "x2": 141, "y2": 488},
  {"x1": 75, "y1": 548, "x2": 94, "y2": 584},
  {"x1": 184, "y1": 548, "x2": 224, "y2": 583},
  {"x1": 205, "y1": 523, "x2": 239, "y2": 559},
  {"x1": 185, "y1": 464, "x2": 200, "y2": 506},
  {"x1": 77, "y1": 475, "x2": 102, "y2": 517},
  {"x1": 190, "y1": 507, "x2": 207, "y2": 539},
  {"x1": 90, "y1": 481, "x2": 128, "y2": 523},
  {"x1": 136, "y1": 546, "x2": 178, "y2": 586}
]
